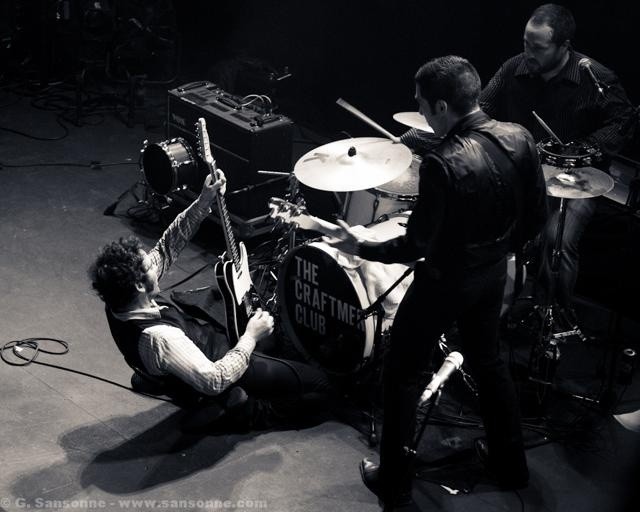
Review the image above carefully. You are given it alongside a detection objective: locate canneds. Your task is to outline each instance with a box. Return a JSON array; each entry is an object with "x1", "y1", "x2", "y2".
[{"x1": 619, "y1": 349, "x2": 636, "y2": 384}]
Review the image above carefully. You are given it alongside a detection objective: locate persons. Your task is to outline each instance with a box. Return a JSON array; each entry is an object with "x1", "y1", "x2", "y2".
[
  {"x1": 474, "y1": 4, "x2": 631, "y2": 371},
  {"x1": 354, "y1": 56, "x2": 548, "y2": 505},
  {"x1": 88, "y1": 168, "x2": 331, "y2": 438}
]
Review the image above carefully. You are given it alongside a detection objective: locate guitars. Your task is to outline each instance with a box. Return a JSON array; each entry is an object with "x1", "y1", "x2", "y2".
[
  {"x1": 267, "y1": 188, "x2": 527, "y2": 318},
  {"x1": 193, "y1": 117, "x2": 281, "y2": 352}
]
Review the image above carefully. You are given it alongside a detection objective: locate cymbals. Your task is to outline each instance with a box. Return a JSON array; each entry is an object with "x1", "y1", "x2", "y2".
[
  {"x1": 392, "y1": 111, "x2": 436, "y2": 134},
  {"x1": 293, "y1": 137, "x2": 414, "y2": 192},
  {"x1": 541, "y1": 163, "x2": 615, "y2": 199}
]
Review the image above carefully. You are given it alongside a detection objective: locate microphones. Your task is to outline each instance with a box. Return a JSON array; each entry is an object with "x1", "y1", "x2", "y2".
[
  {"x1": 419, "y1": 347, "x2": 465, "y2": 403},
  {"x1": 578, "y1": 57, "x2": 606, "y2": 97}
]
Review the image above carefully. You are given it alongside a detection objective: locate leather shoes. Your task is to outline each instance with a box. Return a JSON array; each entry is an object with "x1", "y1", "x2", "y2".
[{"x1": 360, "y1": 458, "x2": 381, "y2": 494}]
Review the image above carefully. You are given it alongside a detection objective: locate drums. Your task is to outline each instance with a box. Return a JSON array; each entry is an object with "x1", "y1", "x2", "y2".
[
  {"x1": 535, "y1": 134, "x2": 602, "y2": 169},
  {"x1": 143, "y1": 138, "x2": 197, "y2": 196},
  {"x1": 342, "y1": 153, "x2": 423, "y2": 228},
  {"x1": 276, "y1": 208, "x2": 441, "y2": 380}
]
